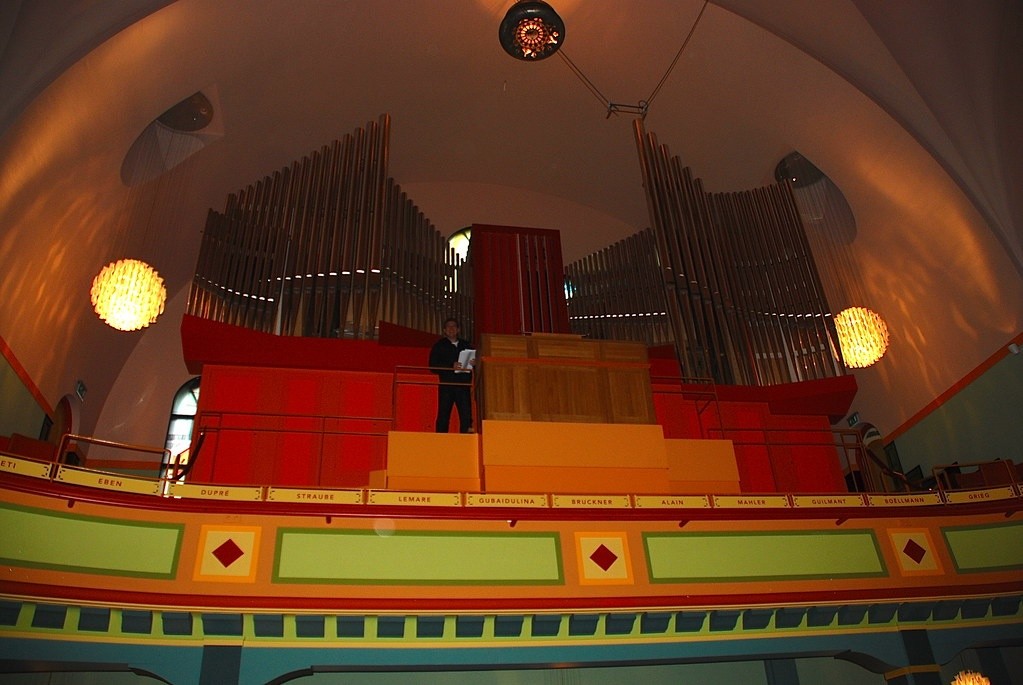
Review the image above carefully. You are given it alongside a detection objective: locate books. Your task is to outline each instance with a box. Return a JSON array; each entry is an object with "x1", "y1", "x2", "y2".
[{"x1": 454, "y1": 349, "x2": 476, "y2": 373}]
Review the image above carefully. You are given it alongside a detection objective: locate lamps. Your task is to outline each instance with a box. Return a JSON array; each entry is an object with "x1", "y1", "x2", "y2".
[
  {"x1": 89, "y1": 91, "x2": 211, "y2": 334},
  {"x1": 773, "y1": 152, "x2": 891, "y2": 372},
  {"x1": 498, "y1": 0, "x2": 567, "y2": 63}
]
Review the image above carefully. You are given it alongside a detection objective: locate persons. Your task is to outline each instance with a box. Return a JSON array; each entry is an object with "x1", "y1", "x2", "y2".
[{"x1": 429, "y1": 317, "x2": 477, "y2": 434}]
